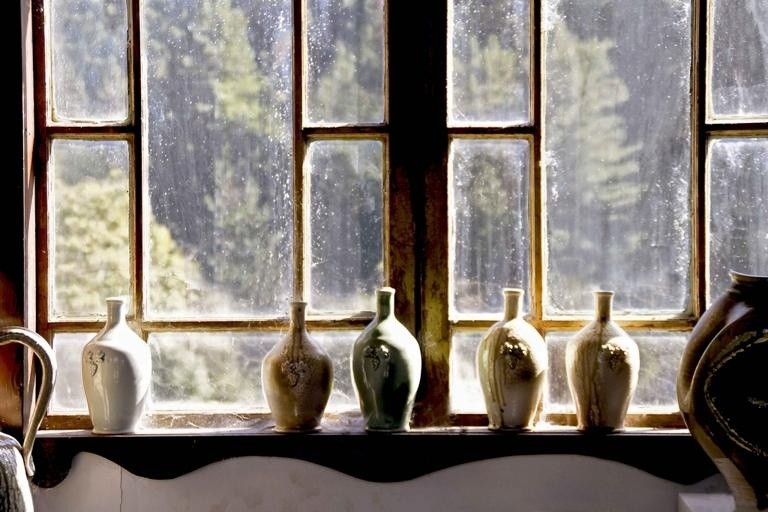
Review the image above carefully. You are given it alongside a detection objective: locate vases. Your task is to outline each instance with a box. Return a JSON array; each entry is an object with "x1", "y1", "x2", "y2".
[
  {"x1": 476, "y1": 288, "x2": 546, "y2": 431},
  {"x1": 83, "y1": 299, "x2": 152, "y2": 434},
  {"x1": 351, "y1": 287, "x2": 422, "y2": 432},
  {"x1": 261, "y1": 302, "x2": 334, "y2": 433},
  {"x1": 565, "y1": 292, "x2": 640, "y2": 434}
]
